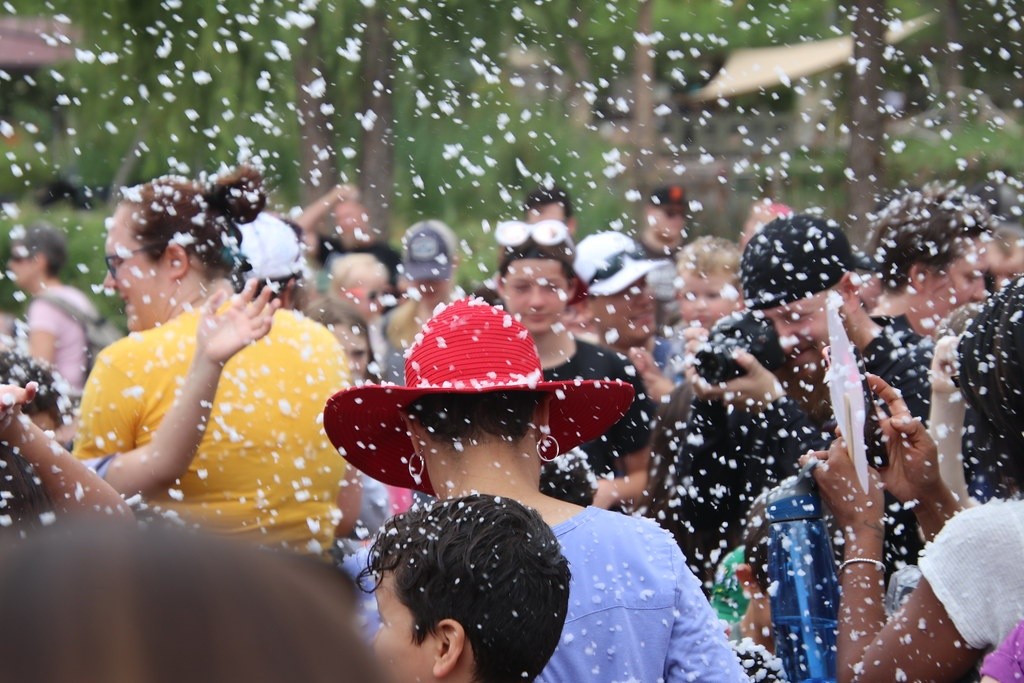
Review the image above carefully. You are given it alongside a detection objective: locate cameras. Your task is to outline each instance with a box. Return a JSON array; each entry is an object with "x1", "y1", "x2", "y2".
[{"x1": 691, "y1": 309, "x2": 786, "y2": 385}]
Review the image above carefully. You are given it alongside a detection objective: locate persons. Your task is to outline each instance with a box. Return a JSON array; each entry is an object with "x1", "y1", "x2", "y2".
[
  {"x1": 464, "y1": 184, "x2": 939, "y2": 683},
  {"x1": 72, "y1": 169, "x2": 362, "y2": 573},
  {"x1": 3, "y1": 226, "x2": 89, "y2": 386},
  {"x1": 1, "y1": 277, "x2": 389, "y2": 683},
  {"x1": 314, "y1": 294, "x2": 752, "y2": 683},
  {"x1": 792, "y1": 267, "x2": 1023, "y2": 683},
  {"x1": 272, "y1": 180, "x2": 463, "y2": 557},
  {"x1": 856, "y1": 173, "x2": 1024, "y2": 342},
  {"x1": 354, "y1": 493, "x2": 573, "y2": 683}
]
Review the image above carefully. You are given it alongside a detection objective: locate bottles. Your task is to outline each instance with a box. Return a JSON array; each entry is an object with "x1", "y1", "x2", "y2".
[{"x1": 765, "y1": 457, "x2": 842, "y2": 683}]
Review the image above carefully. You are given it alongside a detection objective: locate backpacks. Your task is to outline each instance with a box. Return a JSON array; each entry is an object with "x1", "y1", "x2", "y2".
[{"x1": 32, "y1": 294, "x2": 123, "y2": 386}]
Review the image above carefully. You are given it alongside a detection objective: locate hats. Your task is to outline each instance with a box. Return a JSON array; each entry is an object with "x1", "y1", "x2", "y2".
[
  {"x1": 323, "y1": 296, "x2": 634, "y2": 496},
  {"x1": 10, "y1": 222, "x2": 67, "y2": 267},
  {"x1": 742, "y1": 216, "x2": 884, "y2": 310},
  {"x1": 570, "y1": 231, "x2": 670, "y2": 296},
  {"x1": 234, "y1": 211, "x2": 310, "y2": 282},
  {"x1": 650, "y1": 185, "x2": 691, "y2": 213},
  {"x1": 402, "y1": 220, "x2": 457, "y2": 280}
]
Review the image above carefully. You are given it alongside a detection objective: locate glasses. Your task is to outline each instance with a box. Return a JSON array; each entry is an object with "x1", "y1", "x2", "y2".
[
  {"x1": 585, "y1": 242, "x2": 650, "y2": 287},
  {"x1": 658, "y1": 204, "x2": 686, "y2": 219},
  {"x1": 494, "y1": 219, "x2": 576, "y2": 260},
  {"x1": 105, "y1": 239, "x2": 191, "y2": 281}
]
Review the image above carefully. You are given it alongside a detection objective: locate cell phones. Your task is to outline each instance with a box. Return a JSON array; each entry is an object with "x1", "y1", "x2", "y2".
[{"x1": 823, "y1": 345, "x2": 890, "y2": 471}]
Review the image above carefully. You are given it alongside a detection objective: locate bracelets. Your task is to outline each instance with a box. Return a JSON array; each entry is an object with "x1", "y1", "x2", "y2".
[{"x1": 839, "y1": 558, "x2": 888, "y2": 574}]
